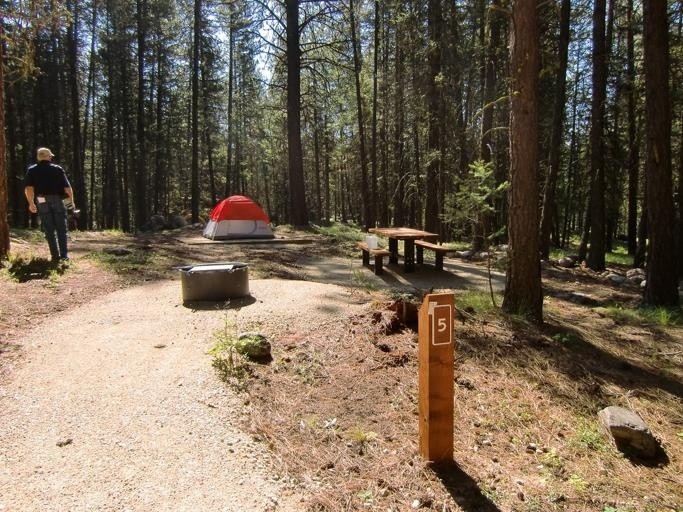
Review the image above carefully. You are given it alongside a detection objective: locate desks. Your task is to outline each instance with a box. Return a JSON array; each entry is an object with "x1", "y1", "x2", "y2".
[{"x1": 368, "y1": 226, "x2": 439, "y2": 273}]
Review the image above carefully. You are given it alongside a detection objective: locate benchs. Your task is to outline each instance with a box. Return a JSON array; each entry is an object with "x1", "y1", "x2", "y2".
[
  {"x1": 358, "y1": 242, "x2": 393, "y2": 275},
  {"x1": 413, "y1": 239, "x2": 457, "y2": 271}
]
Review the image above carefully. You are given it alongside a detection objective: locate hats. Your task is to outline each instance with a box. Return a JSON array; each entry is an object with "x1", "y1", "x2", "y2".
[{"x1": 37, "y1": 148, "x2": 54, "y2": 157}]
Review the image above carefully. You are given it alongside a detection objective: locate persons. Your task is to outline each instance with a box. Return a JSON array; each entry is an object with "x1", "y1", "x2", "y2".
[{"x1": 23, "y1": 147, "x2": 75, "y2": 260}]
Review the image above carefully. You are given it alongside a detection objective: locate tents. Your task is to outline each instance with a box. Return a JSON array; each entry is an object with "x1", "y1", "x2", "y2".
[{"x1": 202, "y1": 195, "x2": 276, "y2": 241}]
